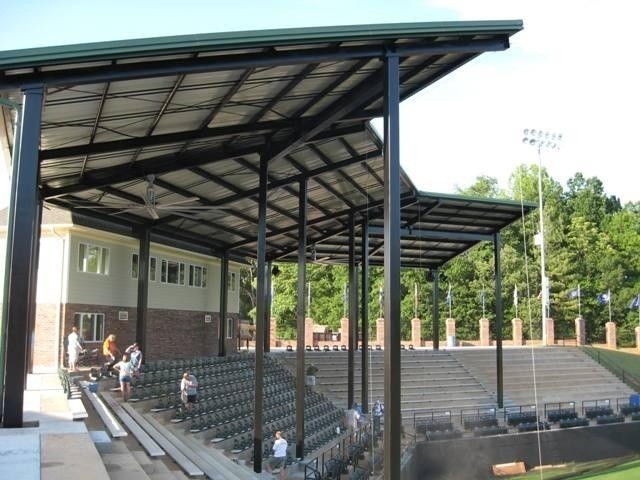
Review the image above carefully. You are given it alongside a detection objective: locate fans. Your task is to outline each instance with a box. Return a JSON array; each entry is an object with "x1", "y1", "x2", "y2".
[
  {"x1": 73, "y1": 174, "x2": 232, "y2": 220},
  {"x1": 306, "y1": 243, "x2": 331, "y2": 266}
]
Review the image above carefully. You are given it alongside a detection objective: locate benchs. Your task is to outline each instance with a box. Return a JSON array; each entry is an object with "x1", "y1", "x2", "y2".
[{"x1": 284, "y1": 345, "x2": 639, "y2": 480}]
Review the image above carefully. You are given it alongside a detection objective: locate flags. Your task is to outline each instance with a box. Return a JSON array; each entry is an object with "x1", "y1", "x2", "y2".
[
  {"x1": 564, "y1": 286, "x2": 579, "y2": 298},
  {"x1": 375, "y1": 281, "x2": 385, "y2": 310},
  {"x1": 627, "y1": 292, "x2": 640, "y2": 311},
  {"x1": 597, "y1": 292, "x2": 613, "y2": 306},
  {"x1": 478, "y1": 286, "x2": 486, "y2": 306},
  {"x1": 446, "y1": 285, "x2": 455, "y2": 307},
  {"x1": 512, "y1": 283, "x2": 519, "y2": 308}
]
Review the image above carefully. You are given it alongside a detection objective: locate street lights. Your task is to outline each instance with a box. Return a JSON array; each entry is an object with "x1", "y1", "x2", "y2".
[{"x1": 523, "y1": 128, "x2": 564, "y2": 345}]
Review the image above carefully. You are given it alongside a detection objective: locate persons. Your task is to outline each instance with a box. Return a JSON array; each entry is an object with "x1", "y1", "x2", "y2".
[
  {"x1": 354, "y1": 401, "x2": 363, "y2": 428},
  {"x1": 67, "y1": 325, "x2": 85, "y2": 370},
  {"x1": 305, "y1": 362, "x2": 321, "y2": 386},
  {"x1": 112, "y1": 354, "x2": 137, "y2": 403},
  {"x1": 125, "y1": 342, "x2": 144, "y2": 373},
  {"x1": 265, "y1": 429, "x2": 289, "y2": 480},
  {"x1": 372, "y1": 399, "x2": 385, "y2": 418},
  {"x1": 342, "y1": 402, "x2": 361, "y2": 445},
  {"x1": 180, "y1": 372, "x2": 199, "y2": 415},
  {"x1": 102, "y1": 333, "x2": 123, "y2": 377}
]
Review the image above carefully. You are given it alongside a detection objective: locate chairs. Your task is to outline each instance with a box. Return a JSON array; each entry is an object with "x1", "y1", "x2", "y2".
[{"x1": 105, "y1": 354, "x2": 345, "y2": 474}]
